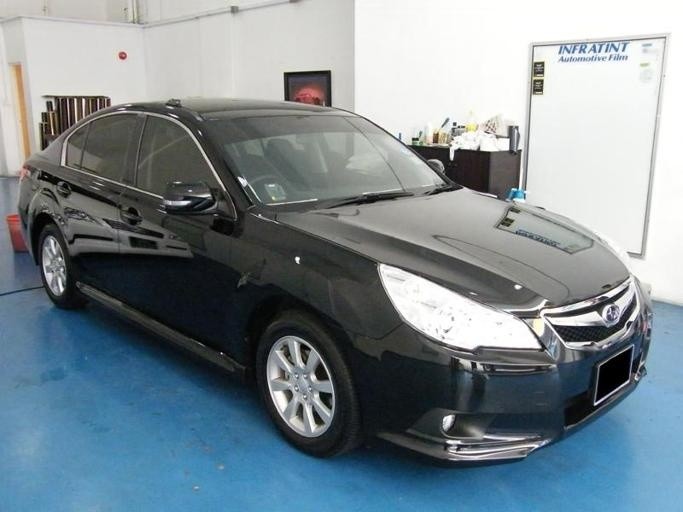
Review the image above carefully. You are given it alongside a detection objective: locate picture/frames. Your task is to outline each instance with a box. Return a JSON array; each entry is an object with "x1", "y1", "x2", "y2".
[{"x1": 283, "y1": 69, "x2": 332, "y2": 107}]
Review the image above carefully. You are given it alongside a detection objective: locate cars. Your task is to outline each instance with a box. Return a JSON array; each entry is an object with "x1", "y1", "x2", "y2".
[{"x1": 16, "y1": 97, "x2": 653, "y2": 468}]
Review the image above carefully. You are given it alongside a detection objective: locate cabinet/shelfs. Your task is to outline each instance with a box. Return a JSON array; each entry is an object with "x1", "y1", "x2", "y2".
[{"x1": 406, "y1": 145, "x2": 522, "y2": 200}]
